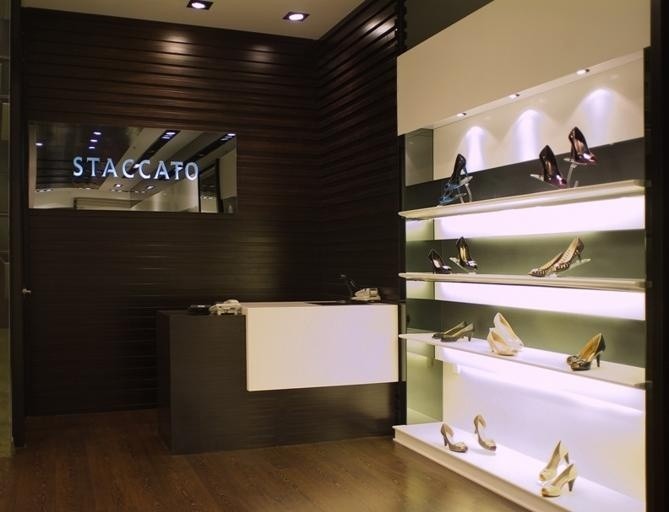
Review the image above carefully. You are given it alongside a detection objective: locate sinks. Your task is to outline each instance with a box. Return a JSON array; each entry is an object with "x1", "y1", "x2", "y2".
[{"x1": 307, "y1": 300, "x2": 353, "y2": 305}]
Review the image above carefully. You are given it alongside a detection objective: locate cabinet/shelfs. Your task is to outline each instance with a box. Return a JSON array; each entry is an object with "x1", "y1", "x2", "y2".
[{"x1": 396, "y1": 0, "x2": 667, "y2": 512}]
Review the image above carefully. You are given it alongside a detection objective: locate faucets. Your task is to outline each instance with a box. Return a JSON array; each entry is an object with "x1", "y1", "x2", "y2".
[{"x1": 336, "y1": 274, "x2": 354, "y2": 296}]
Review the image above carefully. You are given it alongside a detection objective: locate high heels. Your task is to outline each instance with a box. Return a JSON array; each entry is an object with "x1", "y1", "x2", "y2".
[
  {"x1": 569, "y1": 126, "x2": 598, "y2": 163},
  {"x1": 539, "y1": 145, "x2": 567, "y2": 188},
  {"x1": 441, "y1": 424, "x2": 468, "y2": 453},
  {"x1": 567, "y1": 333, "x2": 604, "y2": 370},
  {"x1": 456, "y1": 236, "x2": 478, "y2": 270},
  {"x1": 488, "y1": 313, "x2": 522, "y2": 356},
  {"x1": 542, "y1": 465, "x2": 576, "y2": 498},
  {"x1": 540, "y1": 441, "x2": 569, "y2": 481},
  {"x1": 429, "y1": 249, "x2": 451, "y2": 274},
  {"x1": 473, "y1": 414, "x2": 497, "y2": 451},
  {"x1": 439, "y1": 153, "x2": 468, "y2": 203},
  {"x1": 530, "y1": 237, "x2": 584, "y2": 277},
  {"x1": 432, "y1": 321, "x2": 474, "y2": 341}
]
